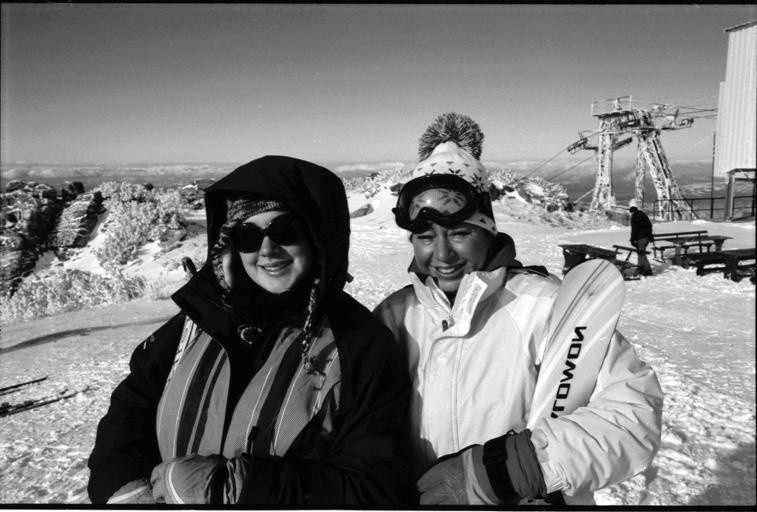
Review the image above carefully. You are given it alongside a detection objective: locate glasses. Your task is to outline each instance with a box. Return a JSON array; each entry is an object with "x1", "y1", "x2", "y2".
[
  {"x1": 395, "y1": 173, "x2": 482, "y2": 233},
  {"x1": 229, "y1": 213, "x2": 308, "y2": 254}
]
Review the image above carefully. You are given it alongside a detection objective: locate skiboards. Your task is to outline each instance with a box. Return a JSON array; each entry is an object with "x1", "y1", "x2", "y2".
[
  {"x1": 526, "y1": 258, "x2": 625, "y2": 505},
  {"x1": 0, "y1": 375, "x2": 78, "y2": 417}
]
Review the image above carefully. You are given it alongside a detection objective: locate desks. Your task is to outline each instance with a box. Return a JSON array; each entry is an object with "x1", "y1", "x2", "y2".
[
  {"x1": 559, "y1": 243, "x2": 623, "y2": 277},
  {"x1": 665, "y1": 235, "x2": 756, "y2": 285}
]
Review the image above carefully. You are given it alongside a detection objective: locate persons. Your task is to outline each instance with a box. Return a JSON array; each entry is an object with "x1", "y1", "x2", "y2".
[
  {"x1": 370, "y1": 115, "x2": 663, "y2": 505},
  {"x1": 92, "y1": 153, "x2": 417, "y2": 508},
  {"x1": 629, "y1": 206, "x2": 653, "y2": 276}
]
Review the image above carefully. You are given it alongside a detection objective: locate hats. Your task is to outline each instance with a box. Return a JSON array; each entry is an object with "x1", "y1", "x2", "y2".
[
  {"x1": 212, "y1": 196, "x2": 323, "y2": 338},
  {"x1": 399, "y1": 139, "x2": 497, "y2": 243}
]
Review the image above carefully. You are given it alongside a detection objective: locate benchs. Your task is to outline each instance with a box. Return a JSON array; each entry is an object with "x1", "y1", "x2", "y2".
[{"x1": 649, "y1": 230, "x2": 715, "y2": 264}]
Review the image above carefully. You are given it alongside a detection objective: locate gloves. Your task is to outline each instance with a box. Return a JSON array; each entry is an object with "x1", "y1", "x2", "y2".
[
  {"x1": 102, "y1": 452, "x2": 251, "y2": 507},
  {"x1": 417, "y1": 427, "x2": 551, "y2": 506}
]
